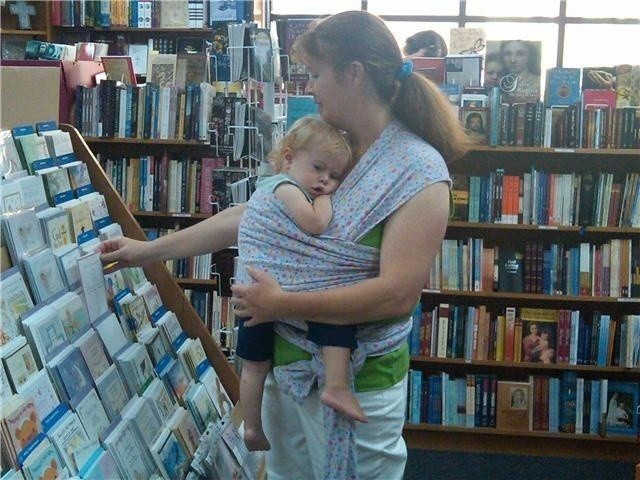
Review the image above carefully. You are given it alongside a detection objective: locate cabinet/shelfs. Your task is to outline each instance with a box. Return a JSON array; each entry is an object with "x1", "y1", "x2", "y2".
[
  {"x1": 402, "y1": 102, "x2": 640, "y2": 460},
  {"x1": 0, "y1": 123, "x2": 268, "y2": 480},
  {"x1": 0, "y1": 0, "x2": 52, "y2": 64},
  {"x1": 54, "y1": 0, "x2": 290, "y2": 380}
]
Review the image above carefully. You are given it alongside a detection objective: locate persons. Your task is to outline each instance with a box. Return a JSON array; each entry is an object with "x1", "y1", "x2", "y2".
[
  {"x1": 93, "y1": 9, "x2": 477, "y2": 480},
  {"x1": 231, "y1": 113, "x2": 370, "y2": 453}
]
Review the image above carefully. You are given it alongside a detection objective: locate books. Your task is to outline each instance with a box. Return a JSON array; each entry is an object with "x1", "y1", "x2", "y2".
[
  {"x1": 405, "y1": 38, "x2": 640, "y2": 149},
  {"x1": 406, "y1": 300, "x2": 639, "y2": 438},
  {"x1": 50, "y1": 0, "x2": 281, "y2": 370},
  {"x1": 423, "y1": 236, "x2": 639, "y2": 298},
  {"x1": 1, "y1": 122, "x2": 266, "y2": 480},
  {"x1": 450, "y1": 164, "x2": 639, "y2": 229}
]
